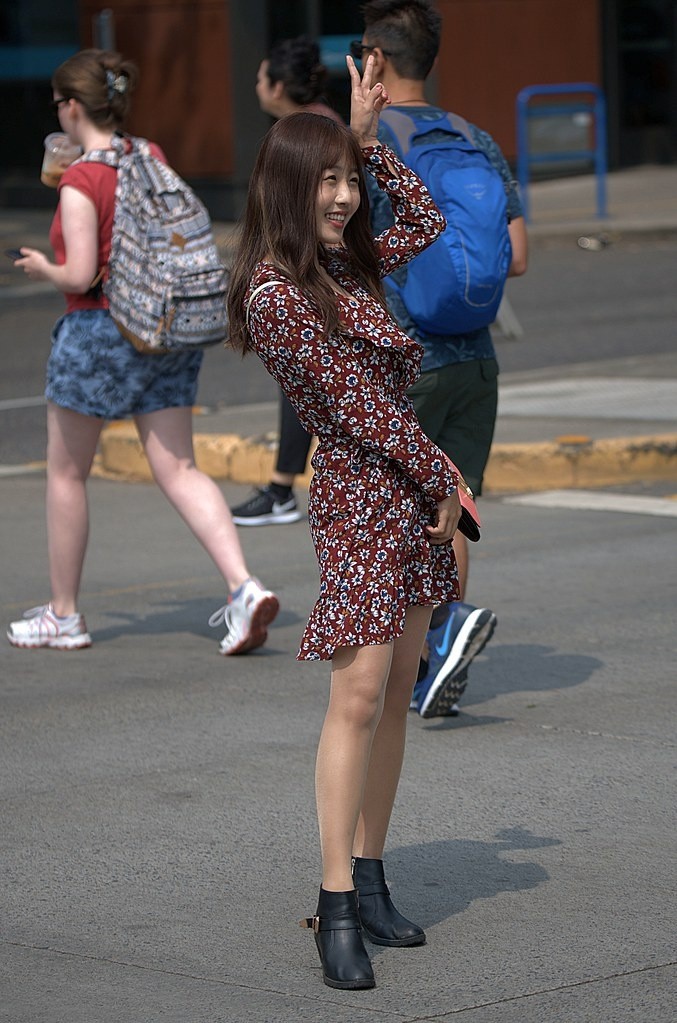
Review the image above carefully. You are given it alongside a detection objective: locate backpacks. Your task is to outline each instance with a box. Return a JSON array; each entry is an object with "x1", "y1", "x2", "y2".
[
  {"x1": 373, "y1": 104, "x2": 508, "y2": 328},
  {"x1": 72, "y1": 135, "x2": 231, "y2": 357}
]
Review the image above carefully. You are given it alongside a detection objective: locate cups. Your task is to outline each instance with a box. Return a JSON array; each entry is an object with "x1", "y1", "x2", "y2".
[{"x1": 40, "y1": 133, "x2": 82, "y2": 187}]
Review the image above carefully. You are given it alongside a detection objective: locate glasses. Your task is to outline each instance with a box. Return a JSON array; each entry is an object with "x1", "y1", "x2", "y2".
[
  {"x1": 349, "y1": 40, "x2": 398, "y2": 60},
  {"x1": 48, "y1": 95, "x2": 61, "y2": 107}
]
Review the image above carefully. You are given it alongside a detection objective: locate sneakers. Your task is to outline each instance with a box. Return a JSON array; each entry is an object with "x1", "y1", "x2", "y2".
[
  {"x1": 209, "y1": 577, "x2": 280, "y2": 657},
  {"x1": 415, "y1": 601, "x2": 497, "y2": 719},
  {"x1": 230, "y1": 484, "x2": 302, "y2": 525},
  {"x1": 408, "y1": 664, "x2": 462, "y2": 714},
  {"x1": 5, "y1": 601, "x2": 91, "y2": 648}
]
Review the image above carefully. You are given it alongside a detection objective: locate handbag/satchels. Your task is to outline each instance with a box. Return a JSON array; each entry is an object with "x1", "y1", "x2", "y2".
[{"x1": 439, "y1": 450, "x2": 482, "y2": 543}]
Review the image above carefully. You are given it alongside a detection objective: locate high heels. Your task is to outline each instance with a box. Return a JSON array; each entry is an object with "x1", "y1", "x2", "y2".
[
  {"x1": 300, "y1": 883, "x2": 374, "y2": 990},
  {"x1": 351, "y1": 856, "x2": 425, "y2": 947}
]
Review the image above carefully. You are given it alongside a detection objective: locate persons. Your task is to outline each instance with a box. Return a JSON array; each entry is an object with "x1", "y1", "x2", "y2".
[
  {"x1": 6, "y1": 49, "x2": 279, "y2": 653},
  {"x1": 231, "y1": 37, "x2": 380, "y2": 526},
  {"x1": 351, "y1": 0, "x2": 527, "y2": 719},
  {"x1": 223, "y1": 56, "x2": 463, "y2": 988}
]
